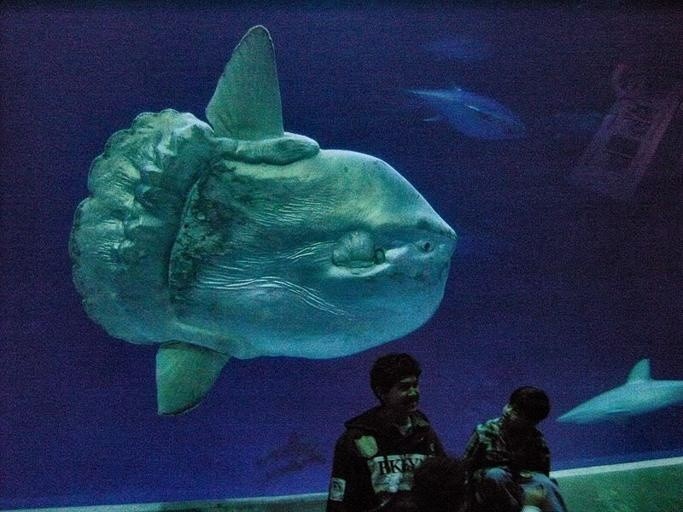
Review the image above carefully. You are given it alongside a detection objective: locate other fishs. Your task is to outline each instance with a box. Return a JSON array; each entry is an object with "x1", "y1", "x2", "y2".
[
  {"x1": 65, "y1": 23, "x2": 458, "y2": 421},
  {"x1": 405, "y1": 84, "x2": 528, "y2": 147}
]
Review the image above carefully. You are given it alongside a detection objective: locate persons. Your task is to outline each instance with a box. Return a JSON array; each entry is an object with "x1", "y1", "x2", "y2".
[
  {"x1": 465, "y1": 464, "x2": 542, "y2": 512},
  {"x1": 365, "y1": 456, "x2": 465, "y2": 512},
  {"x1": 326, "y1": 352, "x2": 450, "y2": 512},
  {"x1": 455, "y1": 385, "x2": 552, "y2": 478}
]
